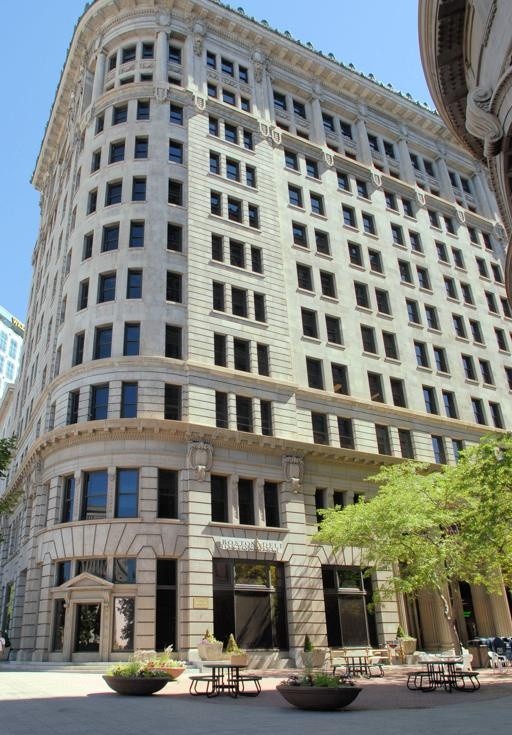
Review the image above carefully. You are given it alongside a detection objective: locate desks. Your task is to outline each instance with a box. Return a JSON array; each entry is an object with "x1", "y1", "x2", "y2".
[
  {"x1": 202, "y1": 661, "x2": 248, "y2": 697},
  {"x1": 417, "y1": 659, "x2": 464, "y2": 693}
]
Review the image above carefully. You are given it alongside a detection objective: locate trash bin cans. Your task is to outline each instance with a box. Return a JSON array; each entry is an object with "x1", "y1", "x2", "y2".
[{"x1": 467, "y1": 637, "x2": 512, "y2": 667}]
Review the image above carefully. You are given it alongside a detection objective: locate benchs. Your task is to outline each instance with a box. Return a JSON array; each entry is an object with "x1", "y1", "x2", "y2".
[
  {"x1": 406, "y1": 668, "x2": 481, "y2": 693},
  {"x1": 188, "y1": 673, "x2": 263, "y2": 698}
]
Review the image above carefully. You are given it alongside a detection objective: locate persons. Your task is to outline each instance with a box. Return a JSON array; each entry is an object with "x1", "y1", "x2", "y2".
[{"x1": 0, "y1": 631, "x2": 10, "y2": 660}]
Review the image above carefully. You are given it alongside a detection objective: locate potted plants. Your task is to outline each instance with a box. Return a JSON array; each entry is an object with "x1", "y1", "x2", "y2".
[
  {"x1": 396, "y1": 624, "x2": 418, "y2": 657},
  {"x1": 198, "y1": 627, "x2": 224, "y2": 659},
  {"x1": 276, "y1": 670, "x2": 362, "y2": 711},
  {"x1": 102, "y1": 653, "x2": 187, "y2": 695},
  {"x1": 300, "y1": 631, "x2": 326, "y2": 667}
]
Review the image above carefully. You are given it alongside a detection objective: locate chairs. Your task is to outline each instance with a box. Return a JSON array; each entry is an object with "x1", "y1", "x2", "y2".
[
  {"x1": 328, "y1": 647, "x2": 389, "y2": 679},
  {"x1": 487, "y1": 650, "x2": 508, "y2": 676}
]
